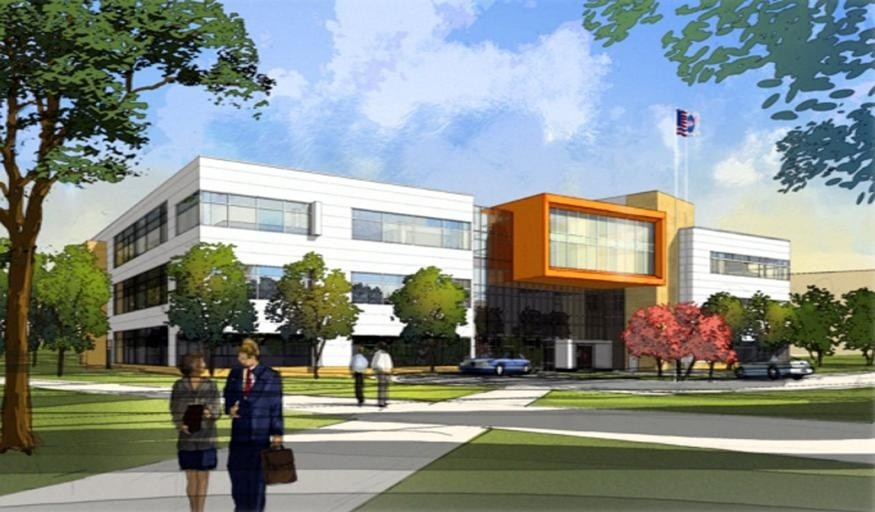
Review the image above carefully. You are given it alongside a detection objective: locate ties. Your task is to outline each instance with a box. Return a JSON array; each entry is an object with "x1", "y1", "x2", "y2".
[{"x1": 243, "y1": 368, "x2": 255, "y2": 391}]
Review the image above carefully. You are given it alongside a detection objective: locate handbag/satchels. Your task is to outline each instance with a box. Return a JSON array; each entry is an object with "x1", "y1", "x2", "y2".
[{"x1": 257, "y1": 440, "x2": 297, "y2": 485}]
